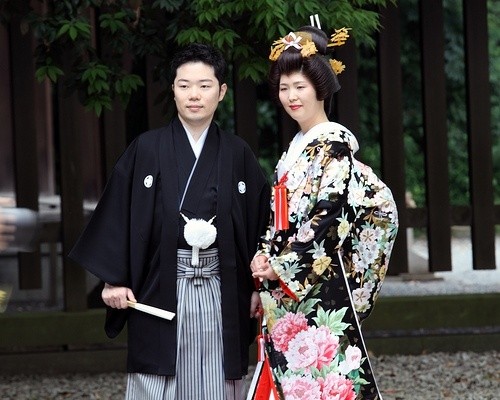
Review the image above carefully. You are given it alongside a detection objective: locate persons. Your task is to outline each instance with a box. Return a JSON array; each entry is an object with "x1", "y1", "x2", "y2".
[
  {"x1": 100, "y1": 47, "x2": 268, "y2": 400},
  {"x1": 250, "y1": 24, "x2": 361, "y2": 398}
]
